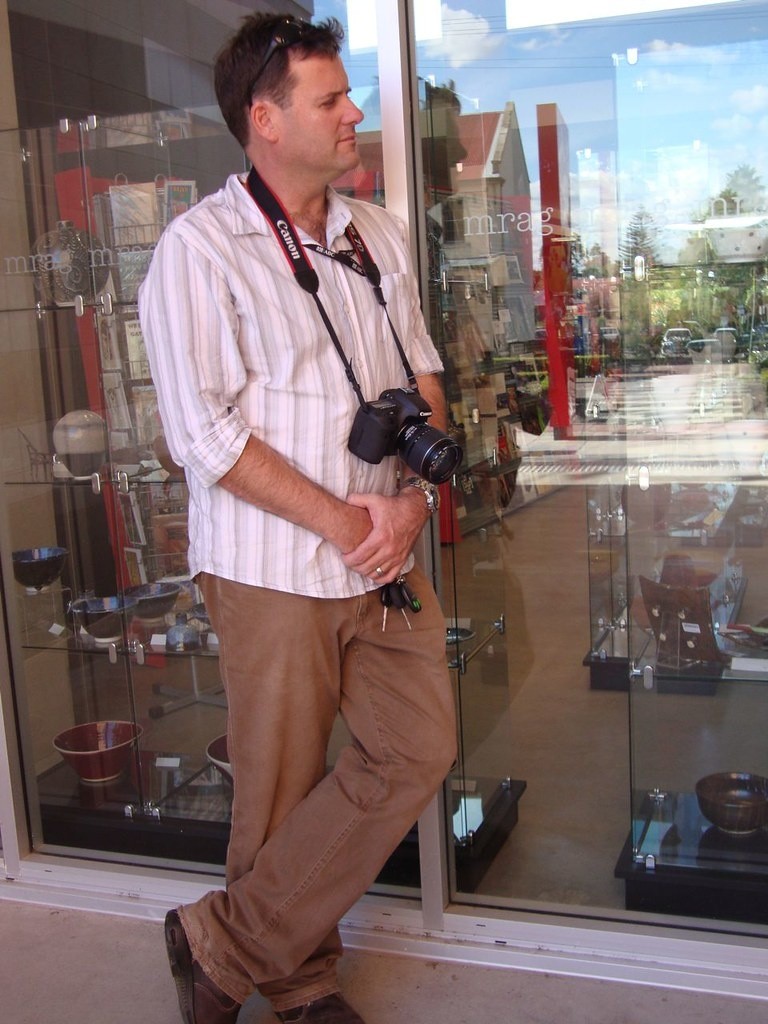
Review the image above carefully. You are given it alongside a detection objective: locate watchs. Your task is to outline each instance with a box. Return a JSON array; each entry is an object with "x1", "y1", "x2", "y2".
[{"x1": 401, "y1": 476, "x2": 441, "y2": 514}]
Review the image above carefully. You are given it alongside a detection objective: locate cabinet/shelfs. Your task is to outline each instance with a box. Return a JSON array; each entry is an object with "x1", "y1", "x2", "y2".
[
  {"x1": 581, "y1": 41, "x2": 768, "y2": 925},
  {"x1": 0, "y1": 103, "x2": 527, "y2": 897}
]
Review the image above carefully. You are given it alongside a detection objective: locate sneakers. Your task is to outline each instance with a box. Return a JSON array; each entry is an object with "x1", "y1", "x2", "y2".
[
  {"x1": 275, "y1": 993, "x2": 367, "y2": 1024},
  {"x1": 164, "y1": 909, "x2": 241, "y2": 1024}
]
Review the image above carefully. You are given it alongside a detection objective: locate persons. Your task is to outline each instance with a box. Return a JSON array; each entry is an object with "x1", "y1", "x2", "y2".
[{"x1": 138, "y1": 10, "x2": 457, "y2": 1023}]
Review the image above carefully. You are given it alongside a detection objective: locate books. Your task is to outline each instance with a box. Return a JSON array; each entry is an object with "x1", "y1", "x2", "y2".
[
  {"x1": 437, "y1": 253, "x2": 575, "y2": 516},
  {"x1": 92, "y1": 178, "x2": 199, "y2": 579}
]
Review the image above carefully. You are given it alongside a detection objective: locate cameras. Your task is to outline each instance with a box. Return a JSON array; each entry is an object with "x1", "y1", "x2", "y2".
[{"x1": 347, "y1": 387, "x2": 464, "y2": 485}]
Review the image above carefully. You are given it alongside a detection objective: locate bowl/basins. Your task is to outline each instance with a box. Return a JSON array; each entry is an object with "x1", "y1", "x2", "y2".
[
  {"x1": 71, "y1": 596, "x2": 134, "y2": 643},
  {"x1": 12, "y1": 546, "x2": 69, "y2": 591},
  {"x1": 189, "y1": 602, "x2": 210, "y2": 626},
  {"x1": 51, "y1": 720, "x2": 145, "y2": 783},
  {"x1": 205, "y1": 733, "x2": 234, "y2": 785},
  {"x1": 696, "y1": 772, "x2": 768, "y2": 835},
  {"x1": 124, "y1": 582, "x2": 181, "y2": 618}
]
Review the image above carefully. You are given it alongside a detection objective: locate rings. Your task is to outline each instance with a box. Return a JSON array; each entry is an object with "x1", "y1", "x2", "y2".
[{"x1": 376, "y1": 567, "x2": 383, "y2": 576}]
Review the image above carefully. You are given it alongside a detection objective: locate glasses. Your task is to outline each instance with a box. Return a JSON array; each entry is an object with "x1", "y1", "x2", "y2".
[{"x1": 246, "y1": 18, "x2": 310, "y2": 111}]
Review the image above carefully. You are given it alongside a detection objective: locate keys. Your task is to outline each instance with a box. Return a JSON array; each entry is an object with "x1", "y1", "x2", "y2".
[{"x1": 380, "y1": 583, "x2": 423, "y2": 632}]
